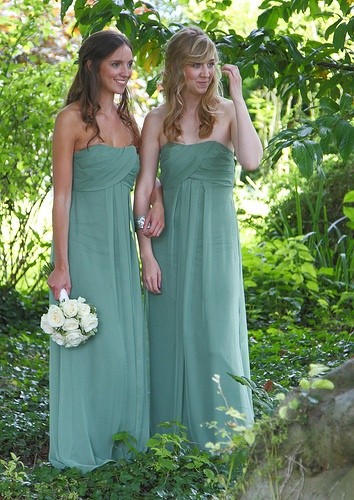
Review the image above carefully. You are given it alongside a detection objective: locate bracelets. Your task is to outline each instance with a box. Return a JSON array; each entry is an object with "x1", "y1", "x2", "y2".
[{"x1": 135, "y1": 216, "x2": 151, "y2": 231}]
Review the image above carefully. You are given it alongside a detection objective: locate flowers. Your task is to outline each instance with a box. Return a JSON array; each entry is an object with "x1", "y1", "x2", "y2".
[{"x1": 39, "y1": 261, "x2": 99, "y2": 349}]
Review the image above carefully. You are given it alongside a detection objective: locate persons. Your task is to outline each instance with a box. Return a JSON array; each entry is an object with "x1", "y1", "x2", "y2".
[
  {"x1": 47, "y1": 29, "x2": 164, "y2": 475},
  {"x1": 133, "y1": 26, "x2": 264, "y2": 460}
]
